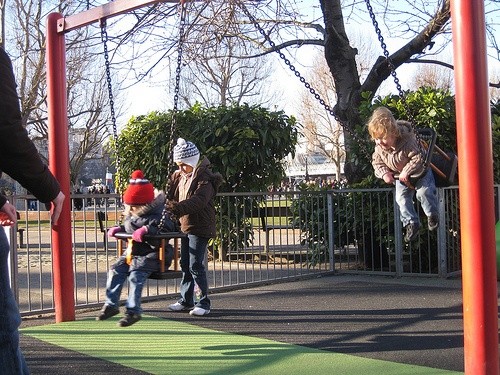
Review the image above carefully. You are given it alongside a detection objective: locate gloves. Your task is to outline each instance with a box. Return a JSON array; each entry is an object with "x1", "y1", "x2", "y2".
[
  {"x1": 108, "y1": 226, "x2": 121, "y2": 240},
  {"x1": 132, "y1": 225, "x2": 148, "y2": 242}
]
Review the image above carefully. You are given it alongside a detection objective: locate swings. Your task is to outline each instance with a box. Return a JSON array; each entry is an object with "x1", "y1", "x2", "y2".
[
  {"x1": 231, "y1": 0, "x2": 460, "y2": 189},
  {"x1": 97, "y1": 0, "x2": 191, "y2": 280}
]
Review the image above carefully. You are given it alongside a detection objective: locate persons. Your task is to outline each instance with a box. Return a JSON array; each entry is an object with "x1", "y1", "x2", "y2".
[
  {"x1": 367, "y1": 106, "x2": 440, "y2": 242},
  {"x1": 0, "y1": 47, "x2": 66, "y2": 375},
  {"x1": 268, "y1": 178, "x2": 348, "y2": 198},
  {"x1": 165, "y1": 138, "x2": 223, "y2": 316},
  {"x1": 96, "y1": 170, "x2": 181, "y2": 327},
  {"x1": 70, "y1": 186, "x2": 110, "y2": 211}
]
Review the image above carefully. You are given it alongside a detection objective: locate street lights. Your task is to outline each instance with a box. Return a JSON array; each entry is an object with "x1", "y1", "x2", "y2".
[{"x1": 304, "y1": 153, "x2": 309, "y2": 182}]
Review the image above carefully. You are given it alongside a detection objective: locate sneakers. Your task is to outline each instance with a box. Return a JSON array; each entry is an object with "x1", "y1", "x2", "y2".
[
  {"x1": 404, "y1": 222, "x2": 421, "y2": 243},
  {"x1": 168, "y1": 301, "x2": 195, "y2": 310},
  {"x1": 95, "y1": 304, "x2": 120, "y2": 321},
  {"x1": 118, "y1": 311, "x2": 141, "y2": 327},
  {"x1": 427, "y1": 217, "x2": 438, "y2": 231},
  {"x1": 189, "y1": 306, "x2": 210, "y2": 315}
]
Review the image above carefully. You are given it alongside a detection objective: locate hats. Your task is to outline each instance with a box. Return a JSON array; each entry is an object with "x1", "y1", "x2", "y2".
[
  {"x1": 122, "y1": 170, "x2": 154, "y2": 206},
  {"x1": 173, "y1": 137, "x2": 200, "y2": 197}
]
